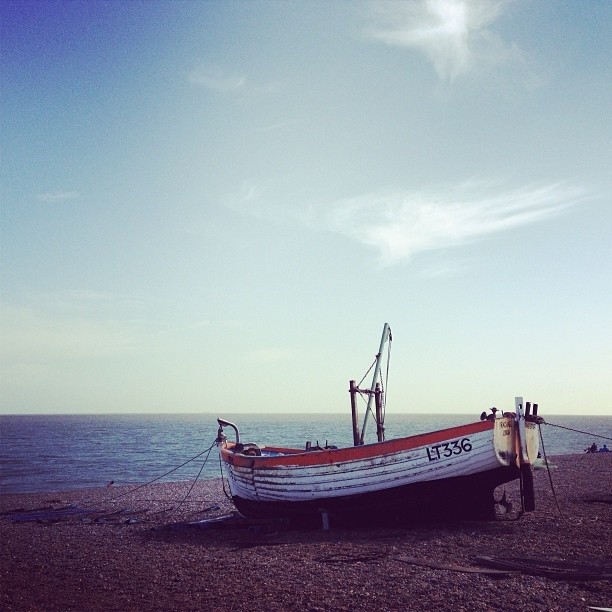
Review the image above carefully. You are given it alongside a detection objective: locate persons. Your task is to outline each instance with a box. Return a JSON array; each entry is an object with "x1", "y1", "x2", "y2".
[
  {"x1": 107, "y1": 480, "x2": 116, "y2": 486},
  {"x1": 600, "y1": 444, "x2": 610, "y2": 451},
  {"x1": 583, "y1": 442, "x2": 598, "y2": 454}
]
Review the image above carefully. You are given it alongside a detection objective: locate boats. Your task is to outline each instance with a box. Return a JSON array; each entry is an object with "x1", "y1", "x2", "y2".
[{"x1": 217, "y1": 322, "x2": 544, "y2": 529}]
